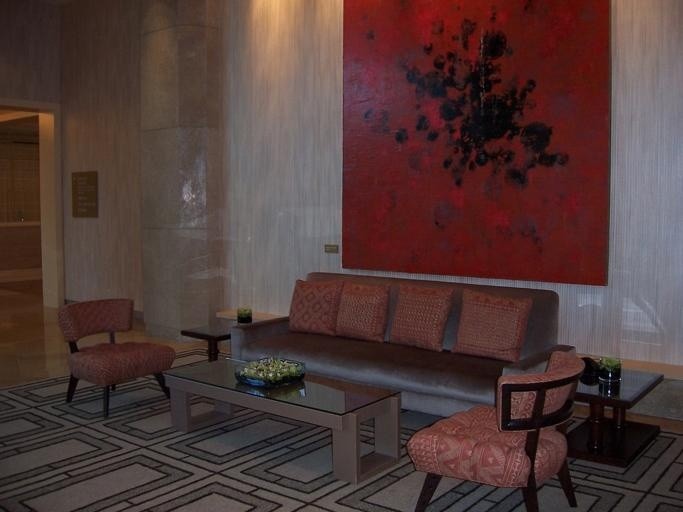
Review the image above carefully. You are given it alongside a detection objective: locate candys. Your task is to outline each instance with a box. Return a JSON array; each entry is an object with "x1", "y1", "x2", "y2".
[{"x1": 242, "y1": 359, "x2": 304, "y2": 383}]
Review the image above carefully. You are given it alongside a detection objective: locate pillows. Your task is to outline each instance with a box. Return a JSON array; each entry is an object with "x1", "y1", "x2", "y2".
[
  {"x1": 384, "y1": 283, "x2": 455, "y2": 352},
  {"x1": 451, "y1": 288, "x2": 533, "y2": 362},
  {"x1": 336, "y1": 282, "x2": 390, "y2": 342},
  {"x1": 289, "y1": 279, "x2": 344, "y2": 337}
]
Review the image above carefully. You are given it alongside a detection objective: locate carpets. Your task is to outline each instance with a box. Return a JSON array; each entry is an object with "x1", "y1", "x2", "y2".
[{"x1": 1, "y1": 348, "x2": 683, "y2": 512}]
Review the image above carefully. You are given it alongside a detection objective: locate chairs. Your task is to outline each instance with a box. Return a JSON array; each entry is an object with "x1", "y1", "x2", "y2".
[
  {"x1": 408, "y1": 350, "x2": 585, "y2": 512},
  {"x1": 58, "y1": 299, "x2": 174, "y2": 418}
]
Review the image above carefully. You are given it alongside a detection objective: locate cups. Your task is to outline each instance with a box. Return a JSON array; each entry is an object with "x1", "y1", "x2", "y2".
[
  {"x1": 599, "y1": 380, "x2": 622, "y2": 398},
  {"x1": 237, "y1": 305, "x2": 252, "y2": 324}
]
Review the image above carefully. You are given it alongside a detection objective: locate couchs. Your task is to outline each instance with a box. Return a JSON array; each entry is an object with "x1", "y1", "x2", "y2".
[{"x1": 231, "y1": 272, "x2": 559, "y2": 418}]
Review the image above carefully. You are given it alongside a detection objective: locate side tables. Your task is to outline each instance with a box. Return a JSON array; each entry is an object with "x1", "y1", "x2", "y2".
[
  {"x1": 181, "y1": 321, "x2": 231, "y2": 362},
  {"x1": 565, "y1": 368, "x2": 664, "y2": 474}
]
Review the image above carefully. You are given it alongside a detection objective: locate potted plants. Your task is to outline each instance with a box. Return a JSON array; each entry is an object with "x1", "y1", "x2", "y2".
[{"x1": 238, "y1": 308, "x2": 252, "y2": 324}]
